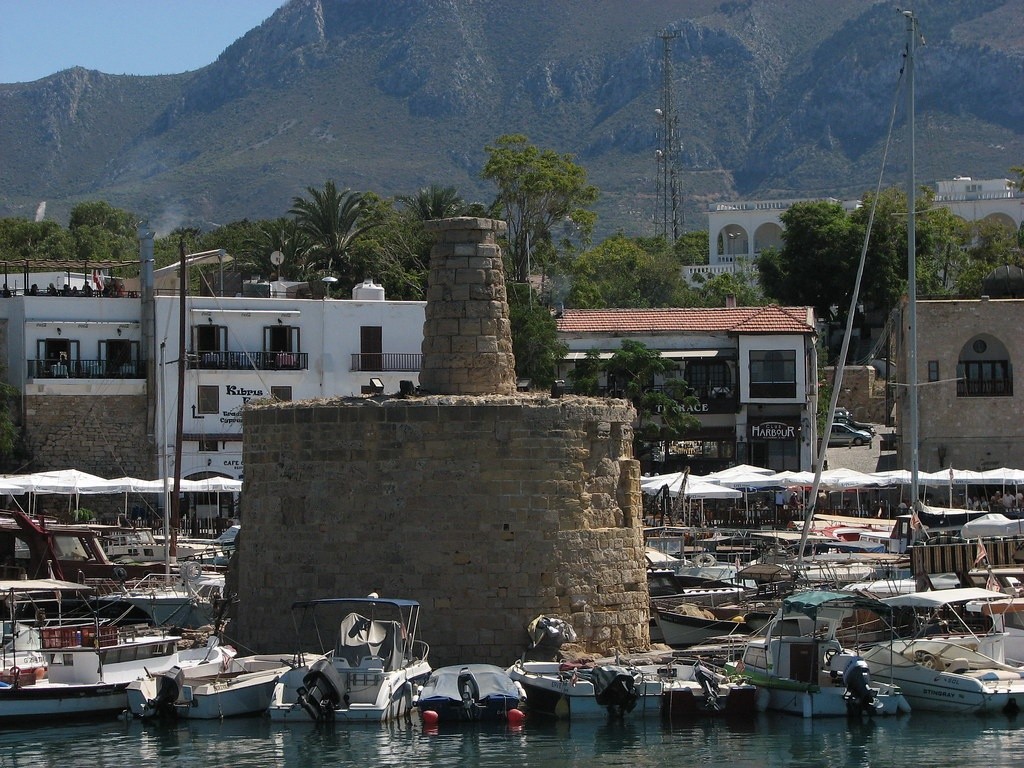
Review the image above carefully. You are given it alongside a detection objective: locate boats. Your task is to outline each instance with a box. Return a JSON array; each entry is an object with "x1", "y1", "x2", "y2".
[
  {"x1": 723, "y1": 591, "x2": 913, "y2": 719},
  {"x1": 645, "y1": 10, "x2": 1024, "y2": 667},
  {"x1": 508, "y1": 658, "x2": 665, "y2": 717},
  {"x1": 0, "y1": 237, "x2": 241, "y2": 679},
  {"x1": 0, "y1": 579, "x2": 238, "y2": 728},
  {"x1": 624, "y1": 657, "x2": 758, "y2": 720},
  {"x1": 127, "y1": 654, "x2": 327, "y2": 723},
  {"x1": 271, "y1": 592, "x2": 432, "y2": 725},
  {"x1": 861, "y1": 587, "x2": 1024, "y2": 716},
  {"x1": 411, "y1": 663, "x2": 527, "y2": 728}
]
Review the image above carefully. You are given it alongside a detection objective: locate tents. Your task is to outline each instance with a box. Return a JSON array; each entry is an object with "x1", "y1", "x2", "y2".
[
  {"x1": 640, "y1": 463, "x2": 1024, "y2": 529},
  {"x1": 1, "y1": 469, "x2": 242, "y2": 533}
]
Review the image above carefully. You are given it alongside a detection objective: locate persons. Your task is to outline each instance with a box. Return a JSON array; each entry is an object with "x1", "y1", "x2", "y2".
[
  {"x1": 786, "y1": 492, "x2": 802, "y2": 510},
  {"x1": 3, "y1": 278, "x2": 128, "y2": 298}
]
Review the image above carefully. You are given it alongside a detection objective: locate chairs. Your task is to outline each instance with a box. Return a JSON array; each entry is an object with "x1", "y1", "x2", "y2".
[
  {"x1": 329, "y1": 656, "x2": 383, "y2": 668},
  {"x1": 943, "y1": 658, "x2": 970, "y2": 674}
]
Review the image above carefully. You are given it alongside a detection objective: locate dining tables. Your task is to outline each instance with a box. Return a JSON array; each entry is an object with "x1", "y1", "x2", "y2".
[
  {"x1": 235, "y1": 353, "x2": 255, "y2": 366},
  {"x1": 203, "y1": 353, "x2": 219, "y2": 367},
  {"x1": 712, "y1": 387, "x2": 730, "y2": 400},
  {"x1": 120, "y1": 365, "x2": 136, "y2": 378},
  {"x1": 86, "y1": 363, "x2": 103, "y2": 375},
  {"x1": 276, "y1": 354, "x2": 296, "y2": 368},
  {"x1": 51, "y1": 364, "x2": 68, "y2": 378}
]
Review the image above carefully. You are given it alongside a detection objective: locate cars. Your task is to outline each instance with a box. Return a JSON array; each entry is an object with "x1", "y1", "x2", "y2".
[
  {"x1": 834, "y1": 414, "x2": 877, "y2": 438},
  {"x1": 827, "y1": 423, "x2": 872, "y2": 447}
]
